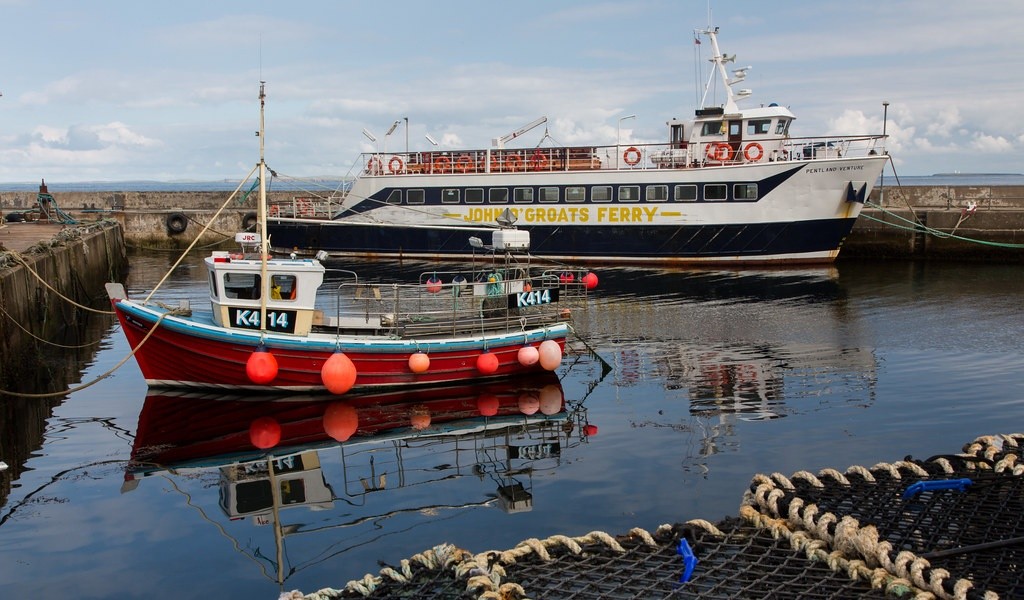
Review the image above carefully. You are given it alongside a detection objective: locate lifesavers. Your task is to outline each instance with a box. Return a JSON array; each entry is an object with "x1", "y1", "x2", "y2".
[
  {"x1": 624, "y1": 147, "x2": 641, "y2": 166},
  {"x1": 744, "y1": 142, "x2": 763, "y2": 161},
  {"x1": 457, "y1": 155, "x2": 473, "y2": 172},
  {"x1": 389, "y1": 157, "x2": 403, "y2": 173},
  {"x1": 243, "y1": 212, "x2": 258, "y2": 232},
  {"x1": 296, "y1": 199, "x2": 307, "y2": 213},
  {"x1": 435, "y1": 156, "x2": 450, "y2": 173},
  {"x1": 368, "y1": 157, "x2": 383, "y2": 174},
  {"x1": 270, "y1": 206, "x2": 279, "y2": 217},
  {"x1": 705, "y1": 142, "x2": 723, "y2": 160},
  {"x1": 505, "y1": 154, "x2": 522, "y2": 170},
  {"x1": 482, "y1": 155, "x2": 497, "y2": 170},
  {"x1": 167, "y1": 212, "x2": 187, "y2": 233},
  {"x1": 530, "y1": 154, "x2": 547, "y2": 169},
  {"x1": 715, "y1": 144, "x2": 733, "y2": 161}
]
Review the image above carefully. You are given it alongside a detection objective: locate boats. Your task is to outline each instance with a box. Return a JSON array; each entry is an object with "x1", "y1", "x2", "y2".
[
  {"x1": 103, "y1": 82, "x2": 574, "y2": 393},
  {"x1": 250, "y1": 1, "x2": 890, "y2": 264},
  {"x1": 118, "y1": 373, "x2": 597, "y2": 591}
]
